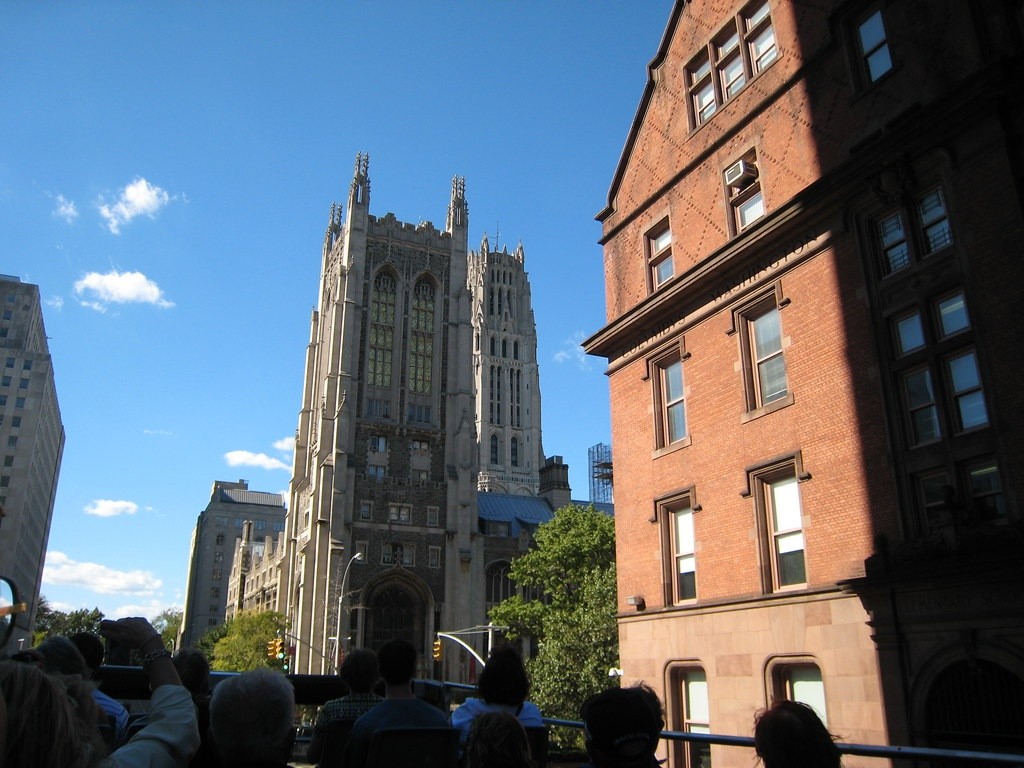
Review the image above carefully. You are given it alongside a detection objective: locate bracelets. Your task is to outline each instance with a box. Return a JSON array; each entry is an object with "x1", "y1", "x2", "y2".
[
  {"x1": 139, "y1": 634, "x2": 161, "y2": 651},
  {"x1": 143, "y1": 648, "x2": 172, "y2": 675}
]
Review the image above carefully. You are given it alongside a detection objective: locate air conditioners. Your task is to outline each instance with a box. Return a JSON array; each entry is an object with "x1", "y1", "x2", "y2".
[{"x1": 724, "y1": 159, "x2": 758, "y2": 188}]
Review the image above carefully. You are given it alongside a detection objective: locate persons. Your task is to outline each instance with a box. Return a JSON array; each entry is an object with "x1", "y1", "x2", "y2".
[
  {"x1": 305, "y1": 647, "x2": 389, "y2": 766},
  {"x1": 66, "y1": 631, "x2": 131, "y2": 754},
  {"x1": 457, "y1": 711, "x2": 540, "y2": 768},
  {"x1": 124, "y1": 645, "x2": 211, "y2": 748},
  {"x1": 0, "y1": 616, "x2": 202, "y2": 768},
  {"x1": 446, "y1": 645, "x2": 546, "y2": 759},
  {"x1": 753, "y1": 701, "x2": 842, "y2": 767},
  {"x1": 578, "y1": 679, "x2": 672, "y2": 768},
  {"x1": 205, "y1": 663, "x2": 297, "y2": 768},
  {"x1": 33, "y1": 634, "x2": 109, "y2": 725},
  {"x1": 339, "y1": 638, "x2": 451, "y2": 768}
]
